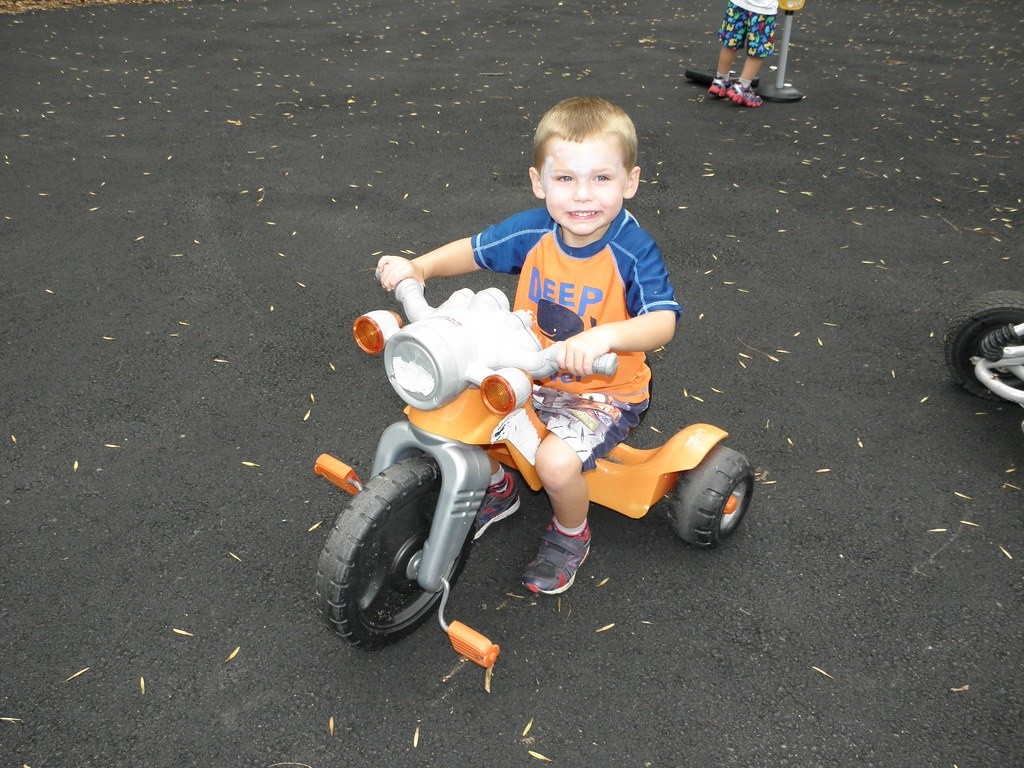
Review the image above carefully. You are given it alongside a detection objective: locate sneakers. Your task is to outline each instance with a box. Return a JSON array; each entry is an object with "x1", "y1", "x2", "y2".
[
  {"x1": 709, "y1": 76, "x2": 762, "y2": 107},
  {"x1": 472, "y1": 473, "x2": 520, "y2": 539},
  {"x1": 522, "y1": 515, "x2": 590, "y2": 594}
]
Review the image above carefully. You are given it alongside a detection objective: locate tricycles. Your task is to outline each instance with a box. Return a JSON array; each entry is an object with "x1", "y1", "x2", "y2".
[{"x1": 313, "y1": 265, "x2": 754, "y2": 668}]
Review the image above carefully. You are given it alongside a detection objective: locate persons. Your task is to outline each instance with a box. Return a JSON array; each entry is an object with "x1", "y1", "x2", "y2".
[
  {"x1": 376, "y1": 95, "x2": 684, "y2": 594},
  {"x1": 708, "y1": 0, "x2": 778, "y2": 107}
]
